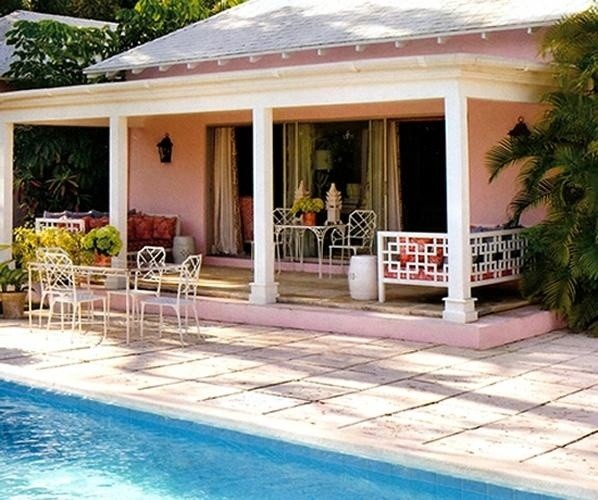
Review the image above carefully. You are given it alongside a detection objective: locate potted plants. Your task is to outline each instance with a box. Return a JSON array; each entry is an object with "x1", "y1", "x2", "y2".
[
  {"x1": 79, "y1": 225, "x2": 124, "y2": 266},
  {"x1": 312, "y1": 132, "x2": 340, "y2": 170},
  {"x1": 1, "y1": 265, "x2": 33, "y2": 320},
  {"x1": 293, "y1": 196, "x2": 324, "y2": 224}
]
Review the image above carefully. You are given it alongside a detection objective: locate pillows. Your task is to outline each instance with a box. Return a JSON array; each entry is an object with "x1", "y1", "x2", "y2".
[{"x1": 127, "y1": 215, "x2": 178, "y2": 243}]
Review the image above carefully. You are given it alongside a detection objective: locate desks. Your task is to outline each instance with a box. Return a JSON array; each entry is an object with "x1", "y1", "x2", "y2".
[
  {"x1": 276, "y1": 223, "x2": 346, "y2": 280},
  {"x1": 24, "y1": 260, "x2": 193, "y2": 346}
]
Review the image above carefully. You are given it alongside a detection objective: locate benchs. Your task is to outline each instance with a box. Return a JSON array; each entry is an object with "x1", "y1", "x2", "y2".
[
  {"x1": 374, "y1": 224, "x2": 531, "y2": 303},
  {"x1": 36, "y1": 208, "x2": 181, "y2": 259}
]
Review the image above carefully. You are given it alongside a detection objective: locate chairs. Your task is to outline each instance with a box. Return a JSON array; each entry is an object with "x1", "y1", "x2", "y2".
[
  {"x1": 328, "y1": 208, "x2": 378, "y2": 280},
  {"x1": 36, "y1": 246, "x2": 205, "y2": 347},
  {"x1": 245, "y1": 208, "x2": 297, "y2": 282}
]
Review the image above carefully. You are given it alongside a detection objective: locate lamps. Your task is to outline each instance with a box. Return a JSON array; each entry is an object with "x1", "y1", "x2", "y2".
[{"x1": 155, "y1": 132, "x2": 174, "y2": 162}]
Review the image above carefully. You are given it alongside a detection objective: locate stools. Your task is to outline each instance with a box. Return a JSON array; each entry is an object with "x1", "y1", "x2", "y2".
[
  {"x1": 347, "y1": 255, "x2": 380, "y2": 302},
  {"x1": 173, "y1": 235, "x2": 196, "y2": 263}
]
